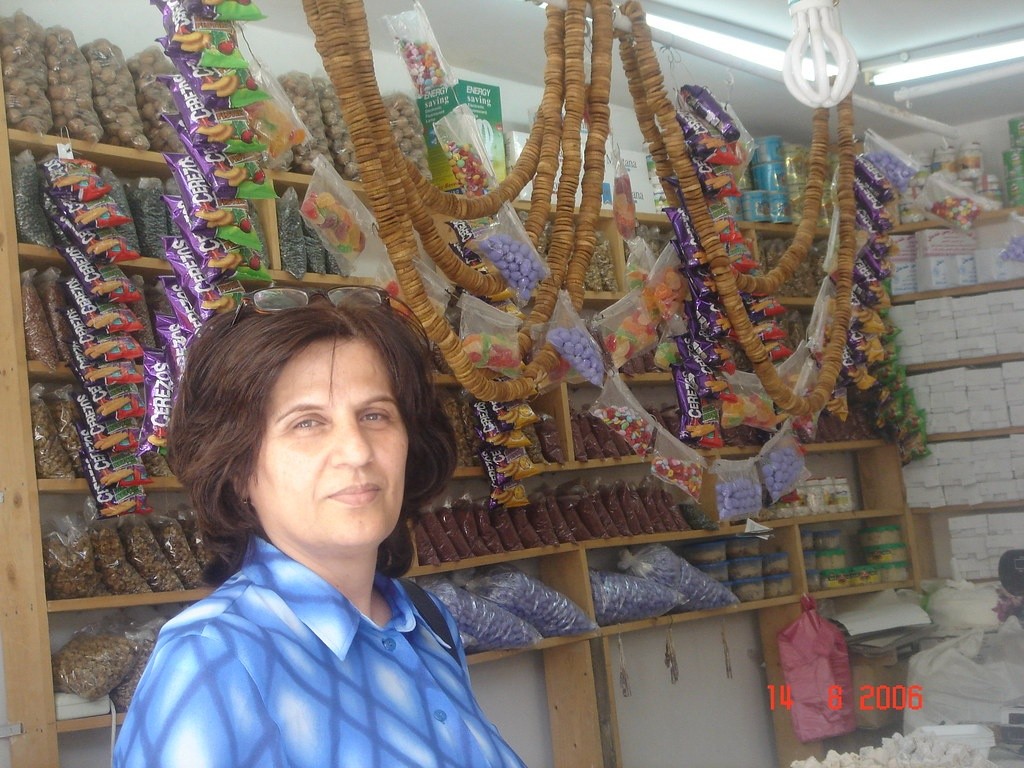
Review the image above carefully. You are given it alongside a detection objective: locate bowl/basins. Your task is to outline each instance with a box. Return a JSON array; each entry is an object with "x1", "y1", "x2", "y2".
[{"x1": 683, "y1": 524, "x2": 909, "y2": 600}]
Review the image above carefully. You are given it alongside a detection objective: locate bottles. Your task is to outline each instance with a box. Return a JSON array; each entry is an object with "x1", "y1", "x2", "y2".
[{"x1": 757, "y1": 477, "x2": 853, "y2": 520}]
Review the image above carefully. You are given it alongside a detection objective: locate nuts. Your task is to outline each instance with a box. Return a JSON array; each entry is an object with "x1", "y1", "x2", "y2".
[
  {"x1": 0, "y1": 12, "x2": 183, "y2": 153},
  {"x1": 277, "y1": 72, "x2": 434, "y2": 184}
]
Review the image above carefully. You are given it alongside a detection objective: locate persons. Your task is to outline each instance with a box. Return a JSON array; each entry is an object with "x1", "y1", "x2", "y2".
[{"x1": 111, "y1": 291, "x2": 528, "y2": 768}]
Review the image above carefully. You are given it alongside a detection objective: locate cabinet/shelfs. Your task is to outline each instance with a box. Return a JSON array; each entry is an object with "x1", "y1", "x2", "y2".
[{"x1": 0, "y1": 86, "x2": 1024, "y2": 768}]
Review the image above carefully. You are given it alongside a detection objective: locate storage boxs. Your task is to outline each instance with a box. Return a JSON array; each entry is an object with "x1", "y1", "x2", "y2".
[
  {"x1": 504, "y1": 118, "x2": 657, "y2": 213},
  {"x1": 417, "y1": 78, "x2": 507, "y2": 191}
]
